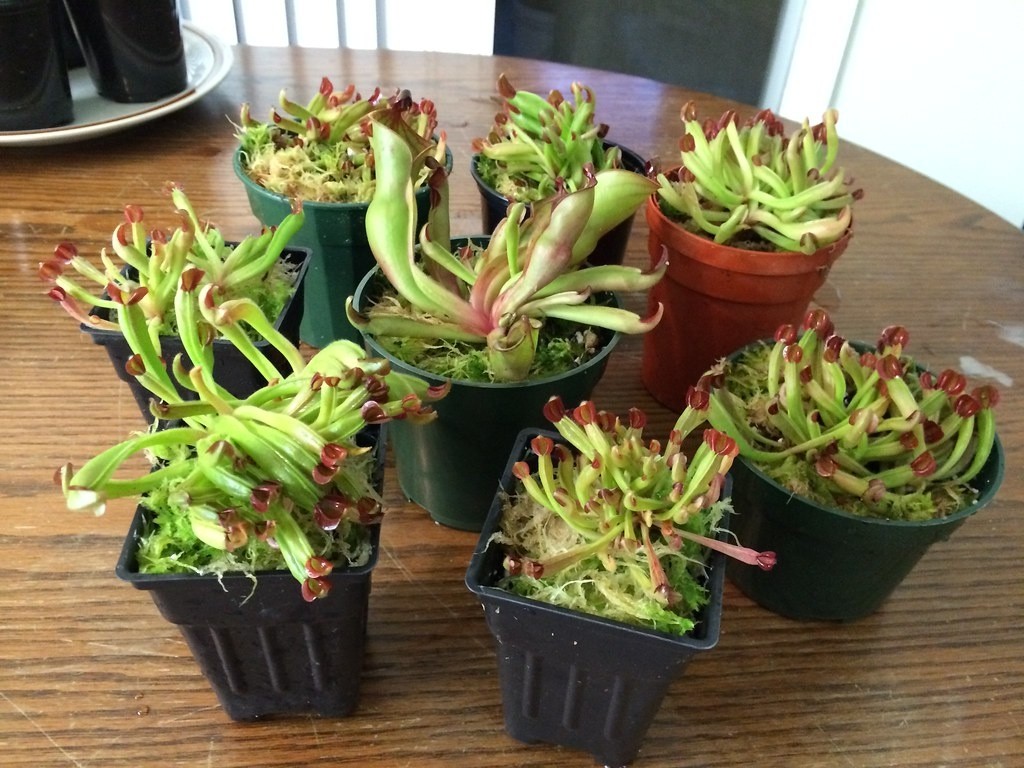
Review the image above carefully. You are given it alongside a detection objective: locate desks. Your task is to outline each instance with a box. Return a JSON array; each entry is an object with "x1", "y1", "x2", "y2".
[{"x1": 1, "y1": 54, "x2": 1024, "y2": 768}]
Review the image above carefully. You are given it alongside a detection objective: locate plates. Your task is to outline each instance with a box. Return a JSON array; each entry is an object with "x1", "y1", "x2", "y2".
[{"x1": 1, "y1": 18, "x2": 237, "y2": 148}]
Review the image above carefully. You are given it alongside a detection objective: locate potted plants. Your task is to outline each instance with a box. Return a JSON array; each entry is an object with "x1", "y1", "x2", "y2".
[{"x1": 32, "y1": 74, "x2": 1006, "y2": 768}]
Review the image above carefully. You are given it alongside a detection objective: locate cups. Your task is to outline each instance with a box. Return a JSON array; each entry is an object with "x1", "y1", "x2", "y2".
[
  {"x1": 62, "y1": 0, "x2": 188, "y2": 104},
  {"x1": 0, "y1": 0, "x2": 74, "y2": 132}
]
